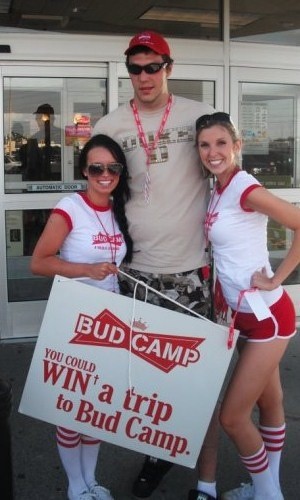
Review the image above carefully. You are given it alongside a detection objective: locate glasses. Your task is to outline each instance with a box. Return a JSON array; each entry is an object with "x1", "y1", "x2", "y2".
[
  {"x1": 86, "y1": 163, "x2": 124, "y2": 178},
  {"x1": 127, "y1": 62, "x2": 169, "y2": 75}
]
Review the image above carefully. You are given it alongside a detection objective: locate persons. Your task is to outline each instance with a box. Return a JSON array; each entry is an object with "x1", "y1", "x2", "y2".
[
  {"x1": 91, "y1": 32, "x2": 218, "y2": 500},
  {"x1": 28, "y1": 105, "x2": 76, "y2": 144},
  {"x1": 196, "y1": 113, "x2": 300, "y2": 500},
  {"x1": 31, "y1": 135, "x2": 132, "y2": 500}
]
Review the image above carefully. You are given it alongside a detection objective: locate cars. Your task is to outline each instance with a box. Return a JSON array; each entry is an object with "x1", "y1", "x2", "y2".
[{"x1": 4, "y1": 155, "x2": 22, "y2": 173}]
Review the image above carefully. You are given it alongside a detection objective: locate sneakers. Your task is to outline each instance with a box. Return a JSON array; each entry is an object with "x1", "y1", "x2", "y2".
[
  {"x1": 221, "y1": 482, "x2": 254, "y2": 500},
  {"x1": 131, "y1": 455, "x2": 174, "y2": 499},
  {"x1": 89, "y1": 482, "x2": 114, "y2": 500},
  {"x1": 67, "y1": 486, "x2": 96, "y2": 500}
]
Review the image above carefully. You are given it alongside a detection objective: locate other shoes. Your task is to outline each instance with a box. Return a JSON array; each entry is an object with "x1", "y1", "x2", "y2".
[{"x1": 187, "y1": 489, "x2": 219, "y2": 500}]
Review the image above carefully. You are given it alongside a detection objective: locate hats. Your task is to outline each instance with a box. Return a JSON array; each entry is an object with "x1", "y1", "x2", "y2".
[
  {"x1": 33, "y1": 104, "x2": 54, "y2": 115},
  {"x1": 124, "y1": 30, "x2": 170, "y2": 56}
]
